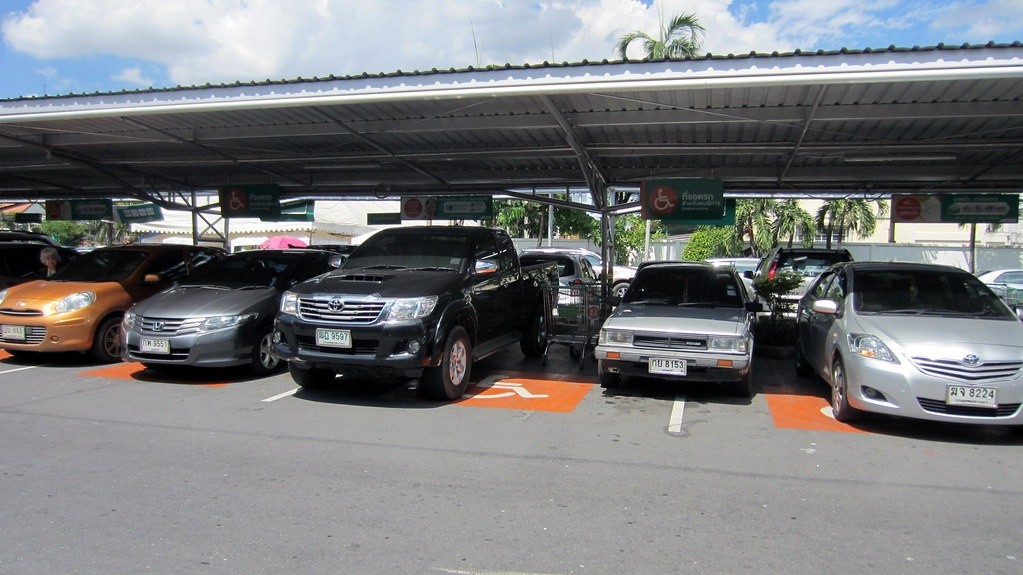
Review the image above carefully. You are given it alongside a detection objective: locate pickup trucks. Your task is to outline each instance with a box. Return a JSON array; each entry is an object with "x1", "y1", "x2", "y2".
[{"x1": 270, "y1": 223, "x2": 560, "y2": 401}]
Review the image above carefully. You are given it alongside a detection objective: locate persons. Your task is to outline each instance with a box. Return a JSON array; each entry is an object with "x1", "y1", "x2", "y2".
[{"x1": 34, "y1": 247, "x2": 63, "y2": 279}]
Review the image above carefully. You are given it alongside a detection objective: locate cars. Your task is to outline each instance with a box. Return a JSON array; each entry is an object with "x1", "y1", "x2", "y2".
[
  {"x1": 970, "y1": 267, "x2": 1023, "y2": 311},
  {"x1": 710, "y1": 256, "x2": 763, "y2": 274},
  {"x1": 522, "y1": 247, "x2": 638, "y2": 300},
  {"x1": 758, "y1": 244, "x2": 854, "y2": 319},
  {"x1": 0, "y1": 244, "x2": 232, "y2": 363},
  {"x1": 793, "y1": 260, "x2": 1023, "y2": 428},
  {"x1": 118, "y1": 248, "x2": 349, "y2": 377},
  {"x1": 0, "y1": 227, "x2": 81, "y2": 293},
  {"x1": 520, "y1": 249, "x2": 600, "y2": 334},
  {"x1": 595, "y1": 260, "x2": 763, "y2": 399}
]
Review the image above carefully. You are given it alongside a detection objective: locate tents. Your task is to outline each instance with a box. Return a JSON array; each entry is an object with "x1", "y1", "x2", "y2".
[{"x1": 130, "y1": 200, "x2": 480, "y2": 249}]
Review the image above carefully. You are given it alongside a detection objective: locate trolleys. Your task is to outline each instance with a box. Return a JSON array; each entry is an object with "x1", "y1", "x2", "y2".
[
  {"x1": 984, "y1": 282, "x2": 1022, "y2": 315},
  {"x1": 537, "y1": 278, "x2": 613, "y2": 370}
]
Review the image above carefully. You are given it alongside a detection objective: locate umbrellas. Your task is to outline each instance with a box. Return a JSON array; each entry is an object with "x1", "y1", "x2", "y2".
[{"x1": 260, "y1": 236, "x2": 308, "y2": 250}]
